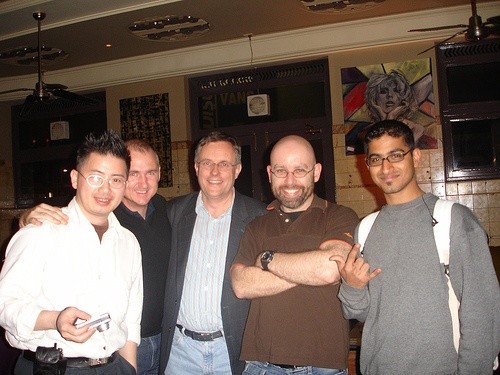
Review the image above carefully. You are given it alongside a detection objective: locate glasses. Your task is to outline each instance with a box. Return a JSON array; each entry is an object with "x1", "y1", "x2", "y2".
[
  {"x1": 270, "y1": 169, "x2": 316, "y2": 179},
  {"x1": 197, "y1": 158, "x2": 239, "y2": 172},
  {"x1": 76, "y1": 170, "x2": 125, "y2": 189},
  {"x1": 365, "y1": 146, "x2": 414, "y2": 169}
]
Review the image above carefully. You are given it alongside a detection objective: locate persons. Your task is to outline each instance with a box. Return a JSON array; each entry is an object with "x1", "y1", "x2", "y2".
[
  {"x1": 0, "y1": 131, "x2": 145, "y2": 375},
  {"x1": 159, "y1": 132, "x2": 273, "y2": 375},
  {"x1": 230, "y1": 135, "x2": 360, "y2": 375},
  {"x1": 19, "y1": 138, "x2": 171, "y2": 375},
  {"x1": 329, "y1": 120, "x2": 500, "y2": 375}
]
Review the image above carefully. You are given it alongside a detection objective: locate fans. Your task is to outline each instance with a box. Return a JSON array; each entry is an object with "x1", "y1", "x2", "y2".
[
  {"x1": 407, "y1": 0, "x2": 500, "y2": 56},
  {"x1": 0, "y1": 11, "x2": 99, "y2": 117}
]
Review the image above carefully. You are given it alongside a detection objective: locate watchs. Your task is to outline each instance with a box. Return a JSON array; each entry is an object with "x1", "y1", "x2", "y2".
[{"x1": 260, "y1": 250, "x2": 274, "y2": 271}]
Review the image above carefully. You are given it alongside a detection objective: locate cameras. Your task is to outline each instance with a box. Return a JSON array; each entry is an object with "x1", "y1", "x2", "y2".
[{"x1": 76, "y1": 315, "x2": 111, "y2": 331}]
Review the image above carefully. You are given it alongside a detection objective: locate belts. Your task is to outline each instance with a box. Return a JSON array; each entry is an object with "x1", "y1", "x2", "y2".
[
  {"x1": 61, "y1": 352, "x2": 116, "y2": 369},
  {"x1": 175, "y1": 323, "x2": 222, "y2": 340}
]
